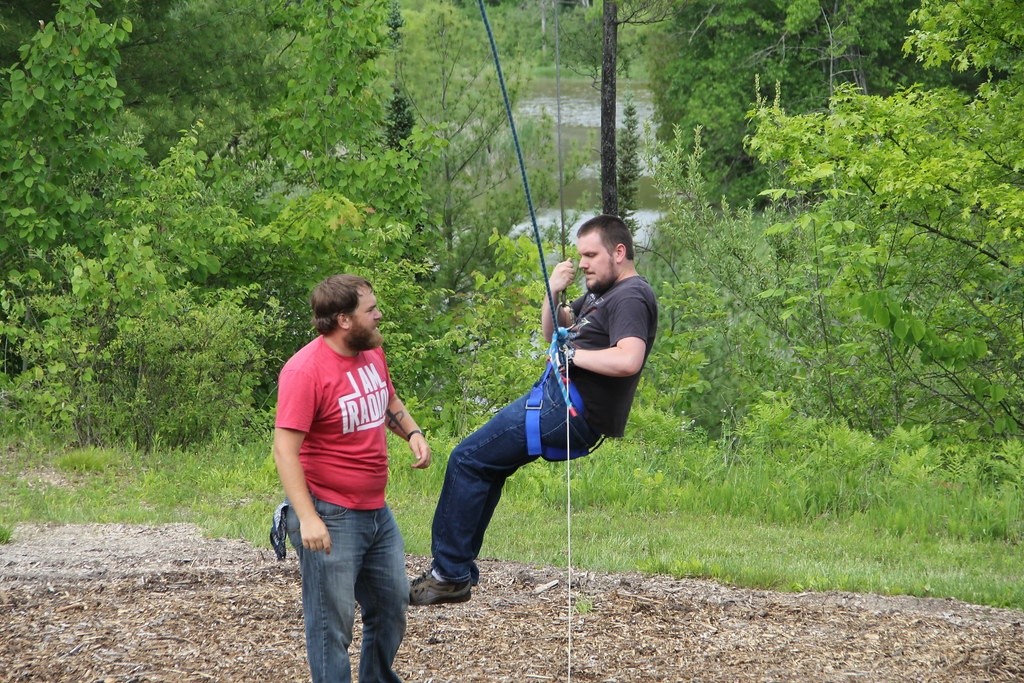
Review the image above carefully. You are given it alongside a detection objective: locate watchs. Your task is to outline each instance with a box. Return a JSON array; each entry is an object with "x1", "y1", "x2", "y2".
[
  {"x1": 406, "y1": 429, "x2": 426, "y2": 440},
  {"x1": 564, "y1": 346, "x2": 575, "y2": 366}
]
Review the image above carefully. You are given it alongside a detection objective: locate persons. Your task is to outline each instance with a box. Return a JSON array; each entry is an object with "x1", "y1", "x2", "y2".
[
  {"x1": 274, "y1": 274, "x2": 430, "y2": 683},
  {"x1": 409, "y1": 216, "x2": 661, "y2": 606}
]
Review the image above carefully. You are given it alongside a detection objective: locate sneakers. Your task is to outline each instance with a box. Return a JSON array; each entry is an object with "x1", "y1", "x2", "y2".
[{"x1": 409, "y1": 568, "x2": 479, "y2": 605}]
